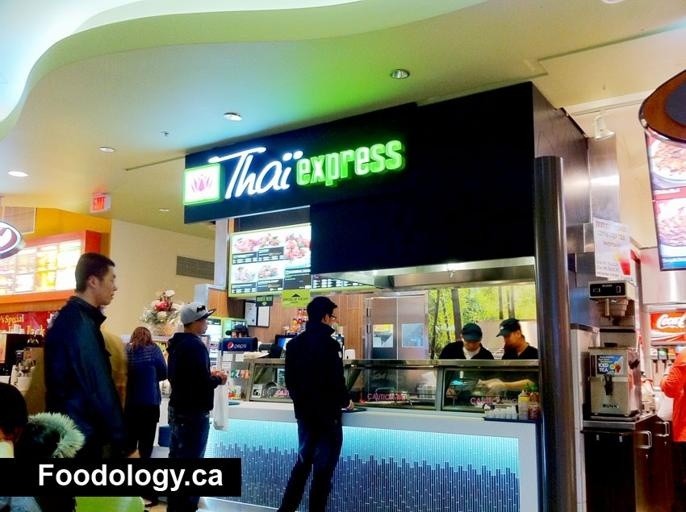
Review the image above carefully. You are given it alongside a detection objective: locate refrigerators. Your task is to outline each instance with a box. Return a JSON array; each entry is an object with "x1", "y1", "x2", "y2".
[{"x1": 365, "y1": 296, "x2": 427, "y2": 390}]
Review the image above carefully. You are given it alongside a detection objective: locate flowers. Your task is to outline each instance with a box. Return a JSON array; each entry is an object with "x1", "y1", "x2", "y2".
[{"x1": 140, "y1": 290, "x2": 178, "y2": 326}]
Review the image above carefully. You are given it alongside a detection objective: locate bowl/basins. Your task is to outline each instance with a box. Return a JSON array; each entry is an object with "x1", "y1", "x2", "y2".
[{"x1": 469, "y1": 387, "x2": 490, "y2": 397}]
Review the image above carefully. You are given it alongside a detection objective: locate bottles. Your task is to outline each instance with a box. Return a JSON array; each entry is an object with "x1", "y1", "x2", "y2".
[
  {"x1": 290, "y1": 308, "x2": 307, "y2": 333},
  {"x1": 517, "y1": 387, "x2": 530, "y2": 420}
]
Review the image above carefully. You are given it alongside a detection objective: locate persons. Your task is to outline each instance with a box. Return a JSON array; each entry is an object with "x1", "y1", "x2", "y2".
[
  {"x1": 659, "y1": 346, "x2": 686, "y2": 511},
  {"x1": 278, "y1": 297, "x2": 356, "y2": 512},
  {"x1": 1, "y1": 381, "x2": 85, "y2": 511},
  {"x1": 476, "y1": 318, "x2": 539, "y2": 399},
  {"x1": 127, "y1": 326, "x2": 168, "y2": 507},
  {"x1": 439, "y1": 323, "x2": 496, "y2": 406},
  {"x1": 44, "y1": 253, "x2": 145, "y2": 496},
  {"x1": 225, "y1": 325, "x2": 250, "y2": 339},
  {"x1": 165, "y1": 302, "x2": 227, "y2": 512}
]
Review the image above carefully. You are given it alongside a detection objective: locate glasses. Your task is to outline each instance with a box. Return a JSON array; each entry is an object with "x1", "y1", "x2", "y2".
[{"x1": 330, "y1": 313, "x2": 337, "y2": 321}]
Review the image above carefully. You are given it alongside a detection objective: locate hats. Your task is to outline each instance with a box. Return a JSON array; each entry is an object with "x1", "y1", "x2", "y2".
[
  {"x1": 180, "y1": 302, "x2": 216, "y2": 325},
  {"x1": 461, "y1": 323, "x2": 482, "y2": 342},
  {"x1": 225, "y1": 324, "x2": 248, "y2": 335},
  {"x1": 496, "y1": 319, "x2": 520, "y2": 337}
]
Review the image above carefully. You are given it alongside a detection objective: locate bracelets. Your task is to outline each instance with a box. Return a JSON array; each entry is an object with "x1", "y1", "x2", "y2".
[
  {"x1": 347, "y1": 400, "x2": 353, "y2": 410},
  {"x1": 662, "y1": 373, "x2": 670, "y2": 376}
]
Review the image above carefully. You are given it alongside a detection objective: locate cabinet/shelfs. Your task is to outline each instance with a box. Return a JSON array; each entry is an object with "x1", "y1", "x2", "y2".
[{"x1": 581, "y1": 414, "x2": 671, "y2": 512}]
